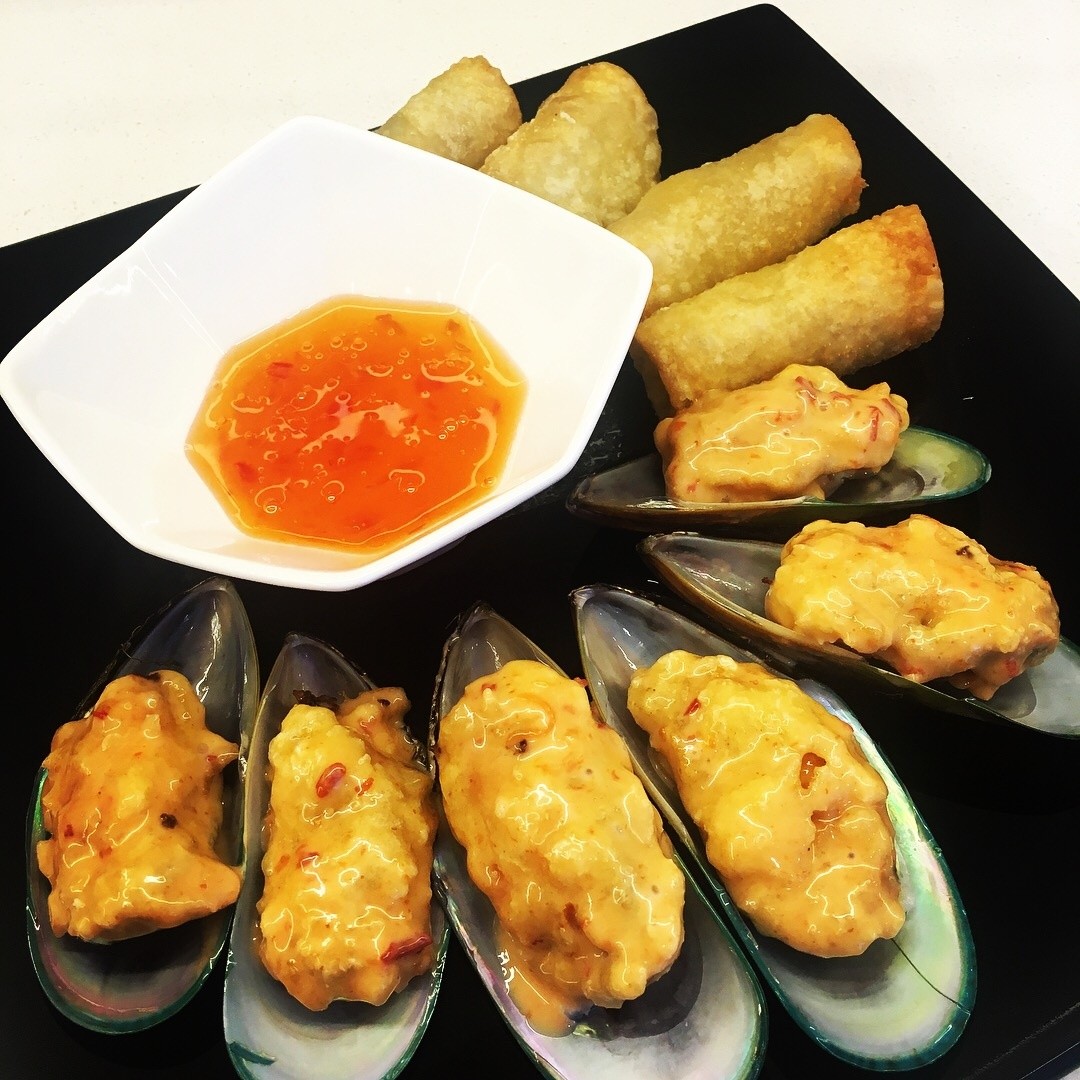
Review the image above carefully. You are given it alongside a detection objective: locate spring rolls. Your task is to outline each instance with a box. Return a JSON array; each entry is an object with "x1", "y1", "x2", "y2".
[
  {"x1": 599, "y1": 113, "x2": 866, "y2": 320},
  {"x1": 476, "y1": 62, "x2": 661, "y2": 224},
  {"x1": 371, "y1": 53, "x2": 522, "y2": 172},
  {"x1": 630, "y1": 202, "x2": 944, "y2": 418}
]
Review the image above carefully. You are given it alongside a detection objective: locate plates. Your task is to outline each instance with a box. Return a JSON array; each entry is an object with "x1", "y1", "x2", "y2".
[{"x1": 0, "y1": 4, "x2": 1080, "y2": 1080}]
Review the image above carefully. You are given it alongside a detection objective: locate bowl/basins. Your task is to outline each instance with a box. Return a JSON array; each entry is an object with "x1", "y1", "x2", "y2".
[{"x1": 2, "y1": 113, "x2": 655, "y2": 592}]
